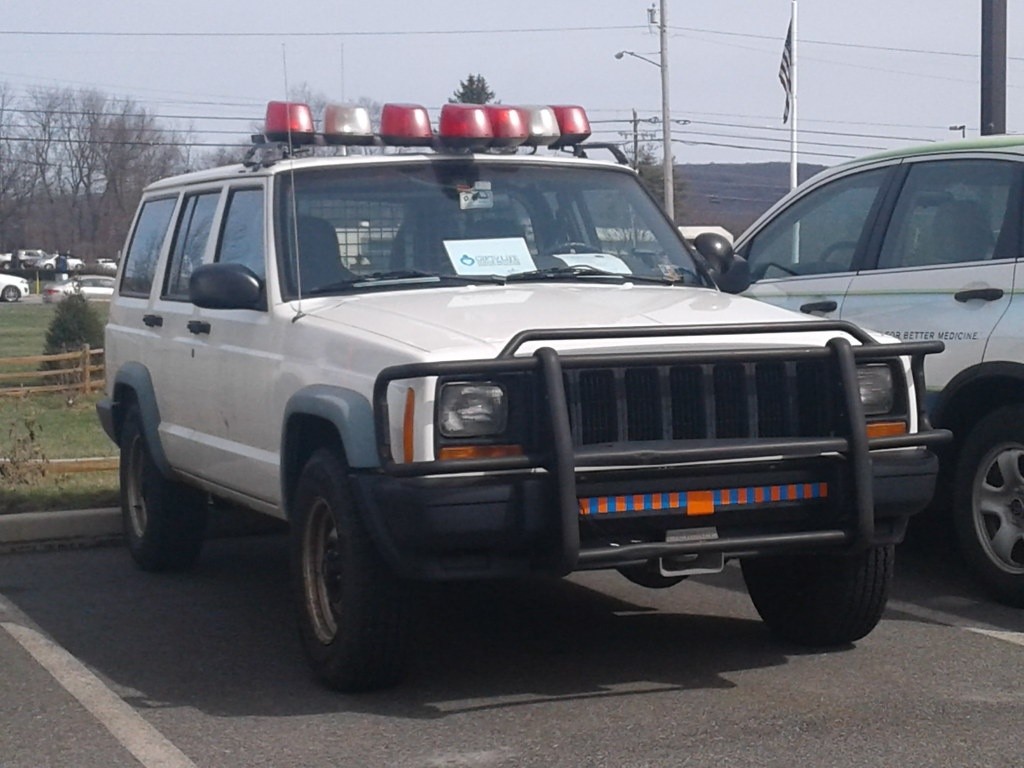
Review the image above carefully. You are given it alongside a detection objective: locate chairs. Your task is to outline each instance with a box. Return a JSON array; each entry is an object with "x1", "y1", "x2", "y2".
[
  {"x1": 293, "y1": 214, "x2": 365, "y2": 293},
  {"x1": 469, "y1": 218, "x2": 530, "y2": 246},
  {"x1": 932, "y1": 199, "x2": 996, "y2": 263}
]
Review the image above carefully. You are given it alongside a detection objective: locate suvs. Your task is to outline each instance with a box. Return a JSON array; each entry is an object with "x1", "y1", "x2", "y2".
[{"x1": 95, "y1": 102, "x2": 954, "y2": 693}]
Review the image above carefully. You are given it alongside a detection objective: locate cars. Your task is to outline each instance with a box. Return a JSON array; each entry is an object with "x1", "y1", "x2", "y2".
[
  {"x1": 43, "y1": 275, "x2": 116, "y2": 304},
  {"x1": 34, "y1": 253, "x2": 87, "y2": 271},
  {"x1": 0, "y1": 250, "x2": 48, "y2": 271},
  {"x1": 96, "y1": 258, "x2": 118, "y2": 271},
  {"x1": 0, "y1": 274, "x2": 30, "y2": 302},
  {"x1": 708, "y1": 132, "x2": 1023, "y2": 608}
]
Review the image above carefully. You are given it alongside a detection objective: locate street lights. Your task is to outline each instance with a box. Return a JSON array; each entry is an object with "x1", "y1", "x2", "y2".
[{"x1": 614, "y1": 50, "x2": 674, "y2": 218}]
